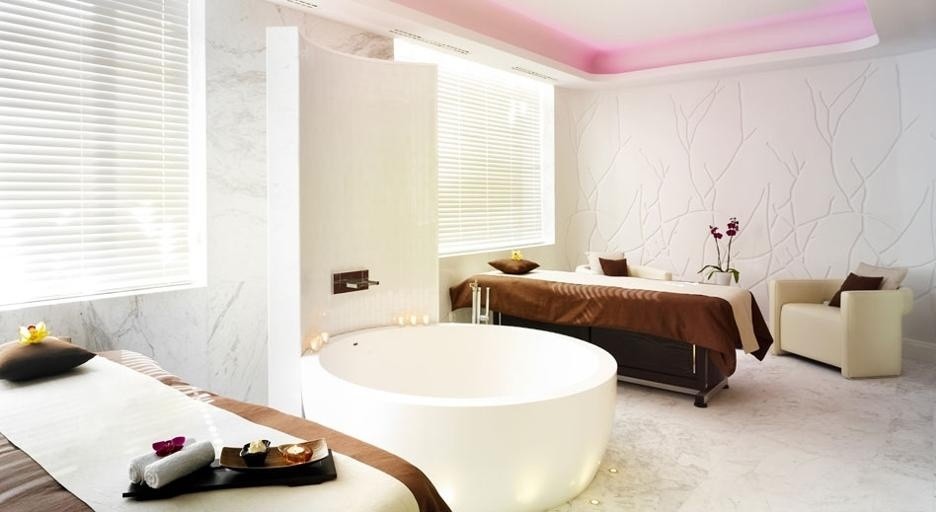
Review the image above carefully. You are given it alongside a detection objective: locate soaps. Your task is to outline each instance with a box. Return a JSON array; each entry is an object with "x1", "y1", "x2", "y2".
[
  {"x1": 245, "y1": 437, "x2": 265, "y2": 452},
  {"x1": 287, "y1": 444, "x2": 305, "y2": 454}
]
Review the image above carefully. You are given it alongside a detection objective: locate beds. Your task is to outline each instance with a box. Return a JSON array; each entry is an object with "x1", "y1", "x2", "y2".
[
  {"x1": 449, "y1": 268, "x2": 774, "y2": 407},
  {"x1": 2, "y1": 320, "x2": 446, "y2": 512}
]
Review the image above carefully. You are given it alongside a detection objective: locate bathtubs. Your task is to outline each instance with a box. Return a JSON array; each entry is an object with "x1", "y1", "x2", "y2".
[{"x1": 300, "y1": 322, "x2": 618, "y2": 510}]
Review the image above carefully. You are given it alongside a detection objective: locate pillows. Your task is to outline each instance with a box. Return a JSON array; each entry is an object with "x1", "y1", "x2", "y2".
[
  {"x1": 828, "y1": 273, "x2": 883, "y2": 309},
  {"x1": 2, "y1": 337, "x2": 97, "y2": 382},
  {"x1": 487, "y1": 258, "x2": 540, "y2": 274},
  {"x1": 599, "y1": 257, "x2": 628, "y2": 276}
]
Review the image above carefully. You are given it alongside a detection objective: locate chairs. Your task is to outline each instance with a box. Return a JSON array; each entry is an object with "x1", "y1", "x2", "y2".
[
  {"x1": 576, "y1": 251, "x2": 673, "y2": 281},
  {"x1": 771, "y1": 262, "x2": 915, "y2": 379}
]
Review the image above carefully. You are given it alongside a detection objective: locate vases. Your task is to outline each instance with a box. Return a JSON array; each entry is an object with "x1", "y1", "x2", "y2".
[{"x1": 711, "y1": 270, "x2": 735, "y2": 285}]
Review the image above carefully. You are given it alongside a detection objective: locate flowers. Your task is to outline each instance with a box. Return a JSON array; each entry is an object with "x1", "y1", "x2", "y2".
[{"x1": 699, "y1": 219, "x2": 760, "y2": 282}]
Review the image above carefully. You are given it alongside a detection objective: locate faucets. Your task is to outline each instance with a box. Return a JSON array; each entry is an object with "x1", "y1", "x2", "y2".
[{"x1": 469, "y1": 279, "x2": 490, "y2": 324}]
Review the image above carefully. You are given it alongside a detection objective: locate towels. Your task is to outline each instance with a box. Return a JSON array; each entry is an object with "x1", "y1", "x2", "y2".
[{"x1": 128, "y1": 441, "x2": 216, "y2": 488}]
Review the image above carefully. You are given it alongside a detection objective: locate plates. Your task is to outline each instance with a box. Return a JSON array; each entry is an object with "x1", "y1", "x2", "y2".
[{"x1": 219, "y1": 439, "x2": 330, "y2": 470}]
